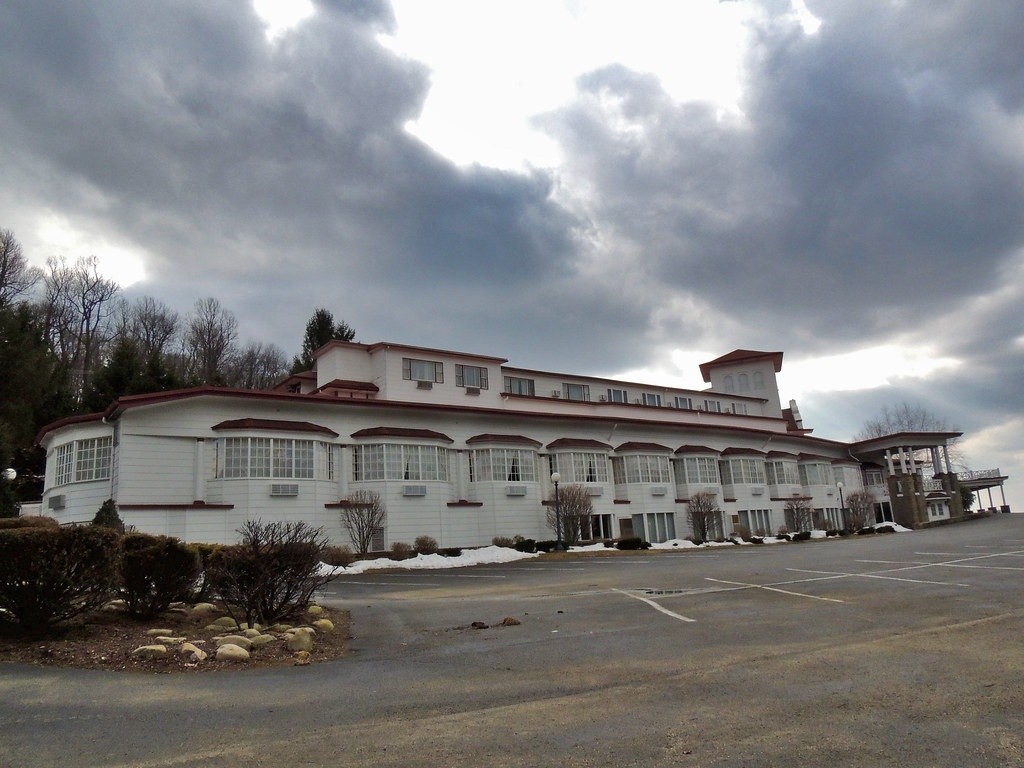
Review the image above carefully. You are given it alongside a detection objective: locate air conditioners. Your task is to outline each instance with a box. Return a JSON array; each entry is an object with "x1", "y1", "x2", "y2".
[
  {"x1": 552, "y1": 390, "x2": 561, "y2": 396},
  {"x1": 600, "y1": 395, "x2": 608, "y2": 400}
]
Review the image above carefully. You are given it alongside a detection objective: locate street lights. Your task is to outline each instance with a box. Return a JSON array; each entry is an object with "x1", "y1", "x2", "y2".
[
  {"x1": 837, "y1": 482, "x2": 852, "y2": 536},
  {"x1": 551, "y1": 472, "x2": 565, "y2": 551}
]
